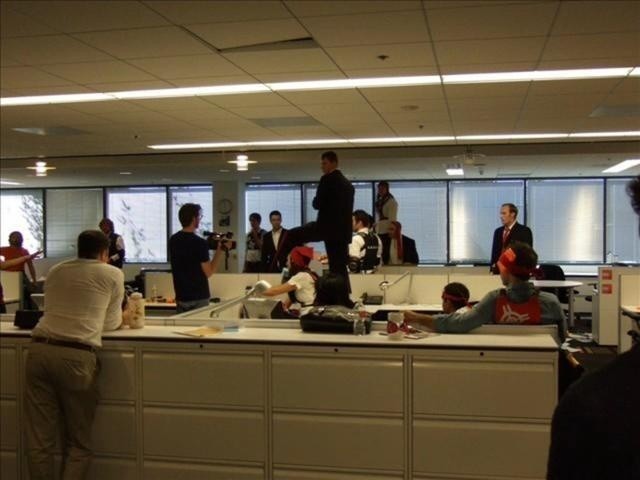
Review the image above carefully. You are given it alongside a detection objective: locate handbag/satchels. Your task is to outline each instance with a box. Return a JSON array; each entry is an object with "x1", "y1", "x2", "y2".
[{"x1": 299, "y1": 307, "x2": 372, "y2": 334}]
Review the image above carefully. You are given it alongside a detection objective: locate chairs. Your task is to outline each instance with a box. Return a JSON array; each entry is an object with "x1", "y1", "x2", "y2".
[
  {"x1": 244, "y1": 297, "x2": 286, "y2": 319},
  {"x1": 537, "y1": 264, "x2": 580, "y2": 321}
]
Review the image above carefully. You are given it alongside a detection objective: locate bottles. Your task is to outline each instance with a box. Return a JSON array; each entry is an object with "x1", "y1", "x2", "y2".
[
  {"x1": 353, "y1": 319, "x2": 366, "y2": 336},
  {"x1": 151, "y1": 284, "x2": 158, "y2": 303},
  {"x1": 128, "y1": 292, "x2": 146, "y2": 329}
]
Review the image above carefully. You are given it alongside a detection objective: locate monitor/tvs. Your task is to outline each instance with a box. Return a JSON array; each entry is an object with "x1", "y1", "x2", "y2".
[
  {"x1": 243, "y1": 298, "x2": 280, "y2": 319},
  {"x1": 140, "y1": 269, "x2": 175, "y2": 300}
]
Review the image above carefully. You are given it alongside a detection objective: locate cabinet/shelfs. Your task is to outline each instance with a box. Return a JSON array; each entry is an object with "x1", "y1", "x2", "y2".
[
  {"x1": 618, "y1": 274, "x2": 640, "y2": 357},
  {"x1": 1, "y1": 271, "x2": 25, "y2": 314},
  {"x1": 592, "y1": 266, "x2": 639, "y2": 345}
]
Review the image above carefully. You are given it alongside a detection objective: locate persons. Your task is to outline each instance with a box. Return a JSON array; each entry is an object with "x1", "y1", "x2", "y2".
[
  {"x1": 403, "y1": 241, "x2": 571, "y2": 340},
  {"x1": 98, "y1": 217, "x2": 125, "y2": 271},
  {"x1": 169, "y1": 203, "x2": 232, "y2": 313},
  {"x1": 489, "y1": 202, "x2": 535, "y2": 275},
  {"x1": 244, "y1": 179, "x2": 420, "y2": 319},
  {"x1": 0, "y1": 231, "x2": 46, "y2": 314},
  {"x1": 544, "y1": 176, "x2": 640, "y2": 479},
  {"x1": 22, "y1": 229, "x2": 126, "y2": 479},
  {"x1": 277, "y1": 150, "x2": 356, "y2": 307},
  {"x1": 441, "y1": 281, "x2": 473, "y2": 315}
]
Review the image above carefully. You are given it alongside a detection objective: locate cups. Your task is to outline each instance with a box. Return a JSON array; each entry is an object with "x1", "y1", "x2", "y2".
[{"x1": 387, "y1": 312, "x2": 405, "y2": 341}]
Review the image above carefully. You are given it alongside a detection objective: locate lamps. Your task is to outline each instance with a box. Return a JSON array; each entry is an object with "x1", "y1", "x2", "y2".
[{"x1": 211, "y1": 281, "x2": 272, "y2": 316}]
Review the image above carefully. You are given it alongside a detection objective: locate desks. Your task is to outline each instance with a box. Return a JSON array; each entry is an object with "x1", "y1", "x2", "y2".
[
  {"x1": 534, "y1": 278, "x2": 583, "y2": 327},
  {"x1": 142, "y1": 299, "x2": 179, "y2": 314},
  {"x1": 362, "y1": 304, "x2": 444, "y2": 319}
]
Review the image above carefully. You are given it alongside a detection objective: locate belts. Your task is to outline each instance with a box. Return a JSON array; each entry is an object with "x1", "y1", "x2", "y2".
[{"x1": 31, "y1": 337, "x2": 95, "y2": 353}]
